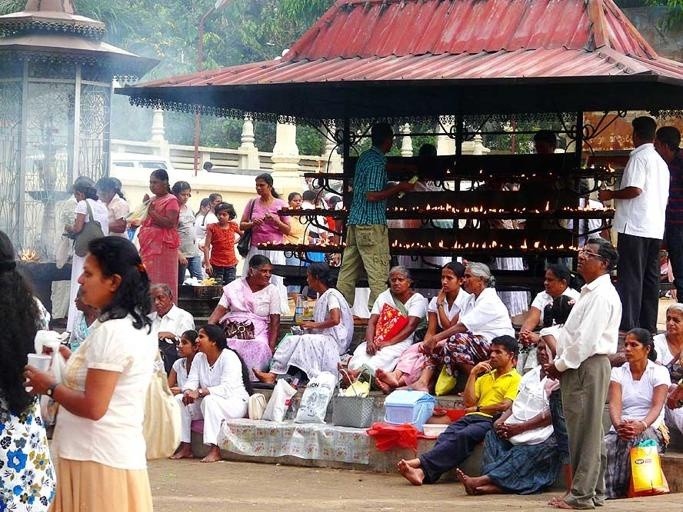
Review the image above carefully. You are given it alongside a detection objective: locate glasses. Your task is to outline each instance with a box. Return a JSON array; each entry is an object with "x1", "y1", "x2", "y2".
[{"x1": 578, "y1": 251, "x2": 607, "y2": 259}]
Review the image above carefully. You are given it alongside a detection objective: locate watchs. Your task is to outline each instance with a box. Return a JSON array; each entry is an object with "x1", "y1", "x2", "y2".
[
  {"x1": 640, "y1": 420, "x2": 647, "y2": 432},
  {"x1": 46, "y1": 383, "x2": 59, "y2": 400},
  {"x1": 197, "y1": 389, "x2": 203, "y2": 398},
  {"x1": 476, "y1": 404, "x2": 482, "y2": 415}
]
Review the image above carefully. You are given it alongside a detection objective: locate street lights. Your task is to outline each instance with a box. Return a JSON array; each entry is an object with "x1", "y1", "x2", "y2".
[{"x1": 193, "y1": 0, "x2": 234, "y2": 176}]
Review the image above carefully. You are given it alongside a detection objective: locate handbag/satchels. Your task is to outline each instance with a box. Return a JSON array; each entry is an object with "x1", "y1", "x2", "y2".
[
  {"x1": 237, "y1": 319, "x2": 255, "y2": 340},
  {"x1": 236, "y1": 198, "x2": 255, "y2": 257},
  {"x1": 217, "y1": 318, "x2": 239, "y2": 338},
  {"x1": 332, "y1": 369, "x2": 374, "y2": 428},
  {"x1": 248, "y1": 393, "x2": 267, "y2": 421}
]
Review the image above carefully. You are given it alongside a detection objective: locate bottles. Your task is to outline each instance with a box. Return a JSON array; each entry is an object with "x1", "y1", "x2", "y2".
[
  {"x1": 303, "y1": 301, "x2": 310, "y2": 317},
  {"x1": 296, "y1": 294, "x2": 304, "y2": 325},
  {"x1": 598, "y1": 179, "x2": 613, "y2": 209}
]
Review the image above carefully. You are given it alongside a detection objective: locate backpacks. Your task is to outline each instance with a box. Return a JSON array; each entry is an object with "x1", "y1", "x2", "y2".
[{"x1": 235, "y1": 195, "x2": 257, "y2": 258}]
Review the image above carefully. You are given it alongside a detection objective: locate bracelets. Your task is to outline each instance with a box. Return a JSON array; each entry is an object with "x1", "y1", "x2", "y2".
[{"x1": 436, "y1": 301, "x2": 445, "y2": 310}]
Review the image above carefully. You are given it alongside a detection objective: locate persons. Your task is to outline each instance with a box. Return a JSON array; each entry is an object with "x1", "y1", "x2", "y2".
[
  {"x1": 95, "y1": 178, "x2": 130, "y2": 238},
  {"x1": 65, "y1": 181, "x2": 108, "y2": 346},
  {"x1": 650, "y1": 125, "x2": 683, "y2": 304},
  {"x1": 192, "y1": 199, "x2": 212, "y2": 238},
  {"x1": 597, "y1": 116, "x2": 671, "y2": 333},
  {"x1": 170, "y1": 181, "x2": 203, "y2": 281},
  {"x1": 327, "y1": 195, "x2": 342, "y2": 244},
  {"x1": 0, "y1": 230, "x2": 57, "y2": 507},
  {"x1": 519, "y1": 264, "x2": 581, "y2": 345},
  {"x1": 539, "y1": 237, "x2": 624, "y2": 510},
  {"x1": 300, "y1": 191, "x2": 329, "y2": 247},
  {"x1": 165, "y1": 328, "x2": 200, "y2": 457},
  {"x1": 149, "y1": 283, "x2": 195, "y2": 375},
  {"x1": 204, "y1": 202, "x2": 245, "y2": 285},
  {"x1": 528, "y1": 132, "x2": 578, "y2": 224},
  {"x1": 455, "y1": 335, "x2": 562, "y2": 495},
  {"x1": 337, "y1": 121, "x2": 417, "y2": 315},
  {"x1": 67, "y1": 286, "x2": 100, "y2": 352},
  {"x1": 205, "y1": 254, "x2": 282, "y2": 383},
  {"x1": 653, "y1": 303, "x2": 683, "y2": 451},
  {"x1": 473, "y1": 186, "x2": 530, "y2": 321},
  {"x1": 238, "y1": 173, "x2": 290, "y2": 313},
  {"x1": 279, "y1": 191, "x2": 309, "y2": 311},
  {"x1": 337, "y1": 264, "x2": 430, "y2": 388},
  {"x1": 540, "y1": 296, "x2": 575, "y2": 503},
  {"x1": 372, "y1": 262, "x2": 471, "y2": 395},
  {"x1": 397, "y1": 335, "x2": 523, "y2": 486},
  {"x1": 601, "y1": 327, "x2": 671, "y2": 499},
  {"x1": 124, "y1": 168, "x2": 180, "y2": 305},
  {"x1": 173, "y1": 324, "x2": 252, "y2": 462},
  {"x1": 199, "y1": 193, "x2": 222, "y2": 254},
  {"x1": 24, "y1": 235, "x2": 157, "y2": 511},
  {"x1": 399, "y1": 260, "x2": 515, "y2": 395},
  {"x1": 410, "y1": 143, "x2": 455, "y2": 230},
  {"x1": 247, "y1": 261, "x2": 353, "y2": 394}
]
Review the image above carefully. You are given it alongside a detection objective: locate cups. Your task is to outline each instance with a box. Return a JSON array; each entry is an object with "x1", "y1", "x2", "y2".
[{"x1": 26, "y1": 353, "x2": 52, "y2": 394}]
